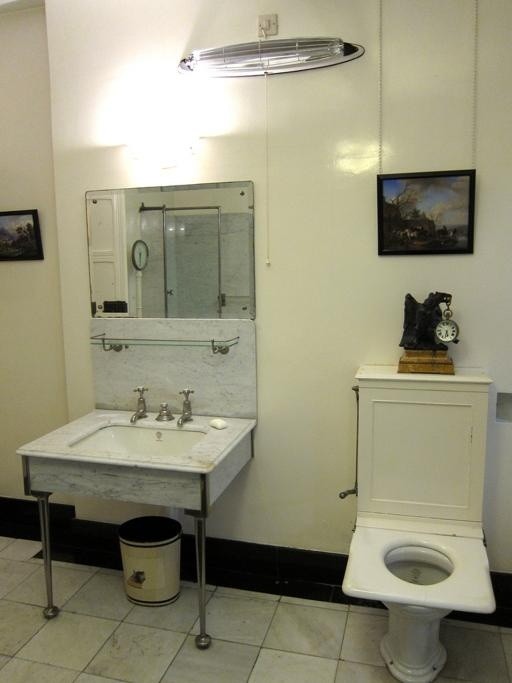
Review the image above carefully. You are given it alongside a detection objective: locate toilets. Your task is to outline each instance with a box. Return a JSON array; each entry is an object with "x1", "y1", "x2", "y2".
[{"x1": 341, "y1": 366, "x2": 495, "y2": 683}]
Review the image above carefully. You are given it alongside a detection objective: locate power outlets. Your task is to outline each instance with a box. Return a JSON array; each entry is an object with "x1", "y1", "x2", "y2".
[{"x1": 258, "y1": 13, "x2": 277, "y2": 36}]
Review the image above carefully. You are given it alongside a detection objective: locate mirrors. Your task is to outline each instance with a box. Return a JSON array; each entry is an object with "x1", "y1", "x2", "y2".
[{"x1": 84, "y1": 179, "x2": 257, "y2": 319}]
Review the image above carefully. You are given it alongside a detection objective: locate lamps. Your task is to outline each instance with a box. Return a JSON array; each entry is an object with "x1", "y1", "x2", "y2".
[{"x1": 180, "y1": 36, "x2": 365, "y2": 77}]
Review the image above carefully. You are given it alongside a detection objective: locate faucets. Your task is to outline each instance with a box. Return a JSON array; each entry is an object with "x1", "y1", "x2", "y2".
[
  {"x1": 130, "y1": 386, "x2": 150, "y2": 424},
  {"x1": 177, "y1": 389, "x2": 195, "y2": 426}
]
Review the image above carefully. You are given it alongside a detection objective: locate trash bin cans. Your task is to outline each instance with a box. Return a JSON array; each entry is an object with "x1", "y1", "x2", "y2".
[{"x1": 120, "y1": 515, "x2": 183, "y2": 607}]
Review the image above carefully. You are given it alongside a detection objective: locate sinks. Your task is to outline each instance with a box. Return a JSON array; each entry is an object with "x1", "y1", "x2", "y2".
[{"x1": 14, "y1": 407, "x2": 257, "y2": 518}]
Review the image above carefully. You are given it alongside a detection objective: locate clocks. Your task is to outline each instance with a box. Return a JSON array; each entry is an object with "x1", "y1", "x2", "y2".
[
  {"x1": 131, "y1": 240, "x2": 150, "y2": 270},
  {"x1": 435, "y1": 320, "x2": 459, "y2": 342}
]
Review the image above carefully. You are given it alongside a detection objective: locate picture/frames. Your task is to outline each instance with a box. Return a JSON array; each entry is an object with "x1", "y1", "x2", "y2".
[
  {"x1": 0, "y1": 208, "x2": 46, "y2": 263},
  {"x1": 375, "y1": 168, "x2": 476, "y2": 256}
]
